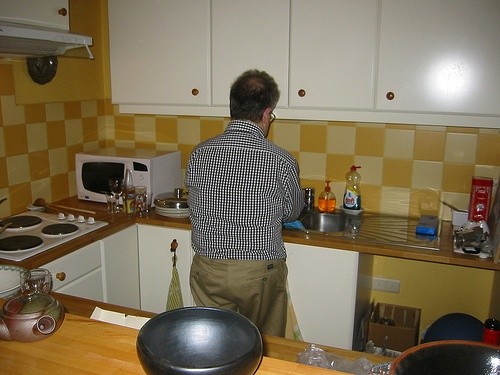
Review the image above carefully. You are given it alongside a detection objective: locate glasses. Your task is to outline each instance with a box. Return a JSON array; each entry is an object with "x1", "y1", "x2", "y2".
[{"x1": 270, "y1": 112, "x2": 276, "y2": 123}]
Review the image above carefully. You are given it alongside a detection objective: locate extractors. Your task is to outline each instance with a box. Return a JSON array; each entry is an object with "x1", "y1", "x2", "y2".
[{"x1": 0, "y1": 0, "x2": 93, "y2": 59}]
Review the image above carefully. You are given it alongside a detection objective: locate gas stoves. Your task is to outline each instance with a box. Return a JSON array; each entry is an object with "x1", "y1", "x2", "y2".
[{"x1": 0, "y1": 210, "x2": 108, "y2": 263}]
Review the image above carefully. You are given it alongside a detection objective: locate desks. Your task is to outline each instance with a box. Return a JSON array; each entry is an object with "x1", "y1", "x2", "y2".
[{"x1": 0, "y1": 298, "x2": 396, "y2": 375}]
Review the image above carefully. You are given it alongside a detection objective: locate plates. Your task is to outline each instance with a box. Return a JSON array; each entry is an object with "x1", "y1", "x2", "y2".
[
  {"x1": 154, "y1": 204, "x2": 190, "y2": 218},
  {"x1": 0, "y1": 264, "x2": 31, "y2": 299}
]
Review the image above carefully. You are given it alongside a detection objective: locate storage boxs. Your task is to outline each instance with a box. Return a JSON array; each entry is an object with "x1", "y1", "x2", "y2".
[{"x1": 368, "y1": 302, "x2": 421, "y2": 353}]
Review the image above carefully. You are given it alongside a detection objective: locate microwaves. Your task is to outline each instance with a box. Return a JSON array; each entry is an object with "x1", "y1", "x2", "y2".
[{"x1": 75, "y1": 146, "x2": 183, "y2": 208}]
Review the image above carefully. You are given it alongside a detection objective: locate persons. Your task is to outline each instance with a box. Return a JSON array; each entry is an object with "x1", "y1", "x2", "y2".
[{"x1": 185, "y1": 69, "x2": 306, "y2": 337}]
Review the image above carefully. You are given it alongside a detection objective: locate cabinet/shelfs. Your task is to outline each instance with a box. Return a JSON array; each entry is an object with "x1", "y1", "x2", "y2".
[
  {"x1": 32, "y1": 226, "x2": 373, "y2": 353},
  {"x1": 107, "y1": 0, "x2": 500, "y2": 129},
  {"x1": 0, "y1": 0, "x2": 70, "y2": 33}
]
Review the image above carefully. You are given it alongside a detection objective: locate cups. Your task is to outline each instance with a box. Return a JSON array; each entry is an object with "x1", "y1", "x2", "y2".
[{"x1": 20, "y1": 268, "x2": 52, "y2": 294}]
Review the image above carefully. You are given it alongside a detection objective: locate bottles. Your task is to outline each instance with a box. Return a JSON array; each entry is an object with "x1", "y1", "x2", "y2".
[
  {"x1": 482, "y1": 318, "x2": 500, "y2": 346},
  {"x1": 108, "y1": 180, "x2": 149, "y2": 217}
]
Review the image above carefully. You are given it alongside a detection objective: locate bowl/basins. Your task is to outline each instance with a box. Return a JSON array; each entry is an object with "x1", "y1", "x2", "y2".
[
  {"x1": 389, "y1": 340, "x2": 500, "y2": 375},
  {"x1": 451, "y1": 209, "x2": 470, "y2": 225},
  {"x1": 136, "y1": 306, "x2": 263, "y2": 375}
]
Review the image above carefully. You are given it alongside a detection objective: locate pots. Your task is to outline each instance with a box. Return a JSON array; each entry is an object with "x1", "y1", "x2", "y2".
[{"x1": 0, "y1": 292, "x2": 65, "y2": 343}]
